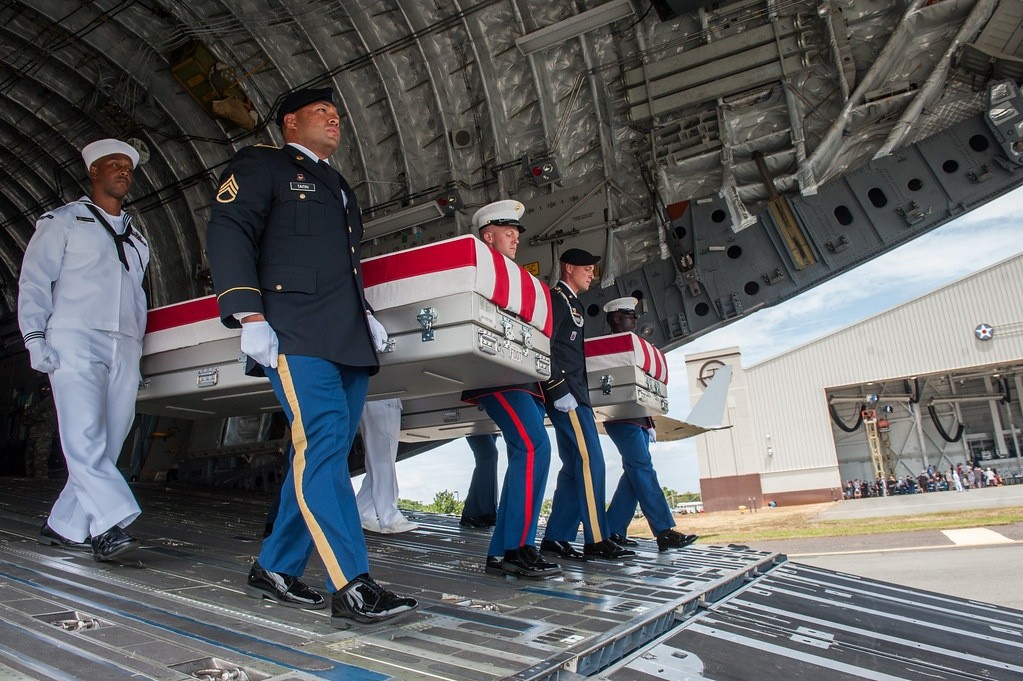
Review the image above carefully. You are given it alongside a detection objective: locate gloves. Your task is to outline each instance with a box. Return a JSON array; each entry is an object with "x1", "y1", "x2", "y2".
[
  {"x1": 241, "y1": 321, "x2": 279, "y2": 368},
  {"x1": 647, "y1": 427, "x2": 657, "y2": 443},
  {"x1": 28, "y1": 340, "x2": 61, "y2": 373},
  {"x1": 366, "y1": 310, "x2": 388, "y2": 352},
  {"x1": 554, "y1": 391, "x2": 578, "y2": 411}
]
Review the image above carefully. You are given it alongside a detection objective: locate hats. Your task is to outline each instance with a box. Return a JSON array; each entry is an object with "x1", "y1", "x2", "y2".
[
  {"x1": 81, "y1": 138, "x2": 141, "y2": 171},
  {"x1": 276, "y1": 86, "x2": 335, "y2": 128},
  {"x1": 560, "y1": 248, "x2": 602, "y2": 266},
  {"x1": 603, "y1": 296, "x2": 638, "y2": 315},
  {"x1": 471, "y1": 200, "x2": 526, "y2": 233}
]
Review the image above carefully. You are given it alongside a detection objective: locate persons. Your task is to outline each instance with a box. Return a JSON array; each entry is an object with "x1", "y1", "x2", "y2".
[
  {"x1": 357, "y1": 400, "x2": 422, "y2": 534},
  {"x1": 205, "y1": 85, "x2": 418, "y2": 621},
  {"x1": 602, "y1": 296, "x2": 700, "y2": 552},
  {"x1": 458, "y1": 200, "x2": 566, "y2": 577},
  {"x1": 17, "y1": 139, "x2": 151, "y2": 565},
  {"x1": 459, "y1": 434, "x2": 500, "y2": 530},
  {"x1": 546, "y1": 248, "x2": 640, "y2": 560},
  {"x1": 841, "y1": 455, "x2": 1003, "y2": 500}
]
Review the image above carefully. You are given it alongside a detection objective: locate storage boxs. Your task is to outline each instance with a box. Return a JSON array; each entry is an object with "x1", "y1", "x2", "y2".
[
  {"x1": 399, "y1": 331, "x2": 670, "y2": 440},
  {"x1": 133, "y1": 235, "x2": 552, "y2": 418}
]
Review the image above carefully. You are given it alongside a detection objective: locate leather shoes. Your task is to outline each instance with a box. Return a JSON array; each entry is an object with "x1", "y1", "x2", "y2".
[
  {"x1": 608, "y1": 533, "x2": 638, "y2": 546},
  {"x1": 459, "y1": 514, "x2": 498, "y2": 529},
  {"x1": 485, "y1": 545, "x2": 562, "y2": 577},
  {"x1": 38, "y1": 520, "x2": 92, "y2": 551},
  {"x1": 331, "y1": 577, "x2": 420, "y2": 629},
  {"x1": 657, "y1": 529, "x2": 699, "y2": 551},
  {"x1": 248, "y1": 561, "x2": 327, "y2": 609},
  {"x1": 361, "y1": 520, "x2": 380, "y2": 532},
  {"x1": 539, "y1": 537, "x2": 587, "y2": 562},
  {"x1": 91, "y1": 526, "x2": 139, "y2": 561},
  {"x1": 582, "y1": 539, "x2": 636, "y2": 559},
  {"x1": 379, "y1": 516, "x2": 419, "y2": 534}
]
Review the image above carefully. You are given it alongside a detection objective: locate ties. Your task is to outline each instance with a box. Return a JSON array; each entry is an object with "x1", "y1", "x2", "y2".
[
  {"x1": 85, "y1": 203, "x2": 145, "y2": 273},
  {"x1": 318, "y1": 158, "x2": 330, "y2": 173}
]
